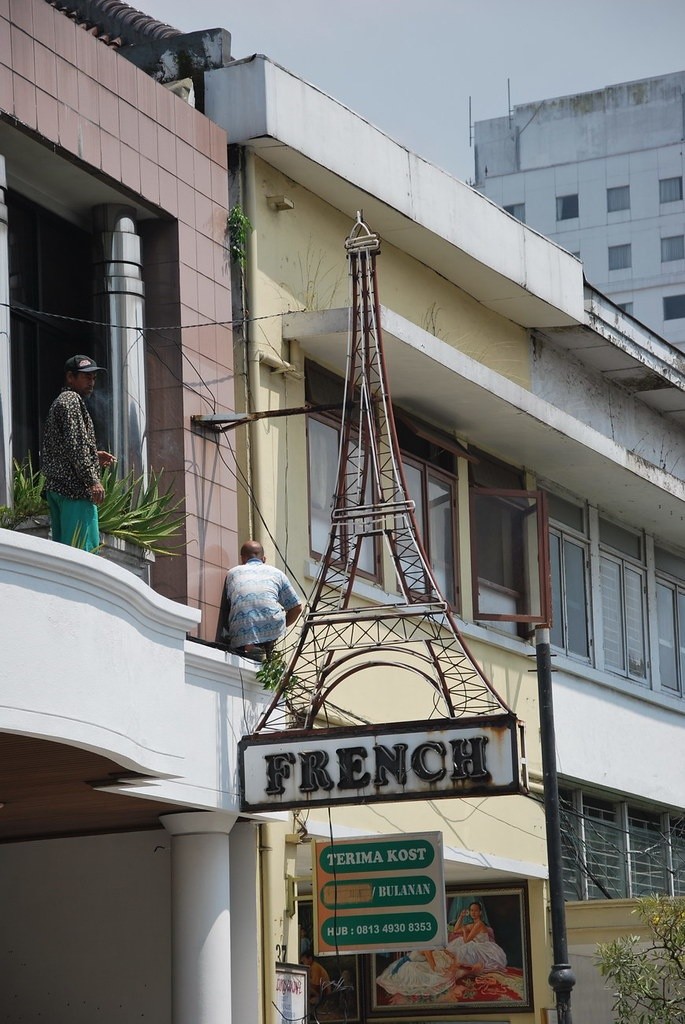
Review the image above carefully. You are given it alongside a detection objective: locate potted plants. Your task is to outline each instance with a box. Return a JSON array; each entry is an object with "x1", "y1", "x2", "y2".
[{"x1": 12, "y1": 449, "x2": 198, "y2": 578}]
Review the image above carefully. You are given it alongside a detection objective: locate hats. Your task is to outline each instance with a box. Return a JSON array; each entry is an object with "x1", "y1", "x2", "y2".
[{"x1": 64, "y1": 355, "x2": 108, "y2": 375}]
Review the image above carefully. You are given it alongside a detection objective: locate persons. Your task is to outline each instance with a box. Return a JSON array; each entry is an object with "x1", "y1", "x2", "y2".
[
  {"x1": 39, "y1": 355, "x2": 117, "y2": 556},
  {"x1": 225, "y1": 540, "x2": 302, "y2": 663}
]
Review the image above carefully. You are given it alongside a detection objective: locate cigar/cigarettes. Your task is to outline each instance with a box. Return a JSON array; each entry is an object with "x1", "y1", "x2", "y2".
[{"x1": 113, "y1": 459, "x2": 117, "y2": 463}]
